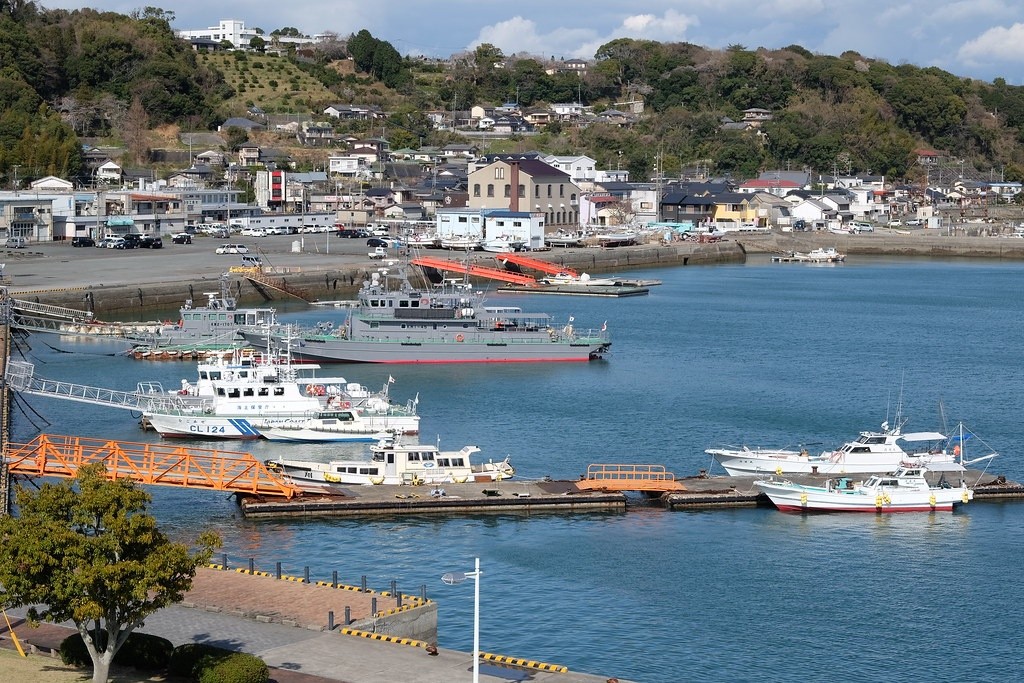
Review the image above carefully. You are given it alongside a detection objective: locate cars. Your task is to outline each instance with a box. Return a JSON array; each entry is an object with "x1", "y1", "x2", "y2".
[
  {"x1": 739, "y1": 222, "x2": 758, "y2": 232},
  {"x1": 366, "y1": 238, "x2": 388, "y2": 247},
  {"x1": 172, "y1": 222, "x2": 398, "y2": 255},
  {"x1": 72, "y1": 237, "x2": 95, "y2": 247},
  {"x1": 793, "y1": 218, "x2": 926, "y2": 235},
  {"x1": 95, "y1": 233, "x2": 163, "y2": 250}
]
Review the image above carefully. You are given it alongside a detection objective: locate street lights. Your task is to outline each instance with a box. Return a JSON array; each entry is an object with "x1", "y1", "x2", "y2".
[{"x1": 441, "y1": 557, "x2": 483, "y2": 683}]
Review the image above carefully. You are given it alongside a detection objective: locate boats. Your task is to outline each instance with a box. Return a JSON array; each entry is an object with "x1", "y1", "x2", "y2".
[
  {"x1": 750, "y1": 457, "x2": 977, "y2": 513},
  {"x1": 263, "y1": 426, "x2": 516, "y2": 486},
  {"x1": 806, "y1": 246, "x2": 846, "y2": 262},
  {"x1": 234, "y1": 222, "x2": 615, "y2": 366},
  {"x1": 134, "y1": 317, "x2": 421, "y2": 443},
  {"x1": 703, "y1": 366, "x2": 998, "y2": 478},
  {"x1": 397, "y1": 221, "x2": 726, "y2": 254}
]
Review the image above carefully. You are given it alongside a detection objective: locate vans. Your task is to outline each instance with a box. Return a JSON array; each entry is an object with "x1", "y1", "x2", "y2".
[
  {"x1": 5, "y1": 236, "x2": 24, "y2": 249},
  {"x1": 240, "y1": 256, "x2": 263, "y2": 268}
]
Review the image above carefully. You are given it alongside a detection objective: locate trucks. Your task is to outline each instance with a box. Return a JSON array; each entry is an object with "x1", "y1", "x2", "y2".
[{"x1": 367, "y1": 247, "x2": 388, "y2": 259}]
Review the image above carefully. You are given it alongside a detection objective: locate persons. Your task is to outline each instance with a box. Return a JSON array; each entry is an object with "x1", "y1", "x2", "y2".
[{"x1": 954, "y1": 443, "x2": 961, "y2": 463}]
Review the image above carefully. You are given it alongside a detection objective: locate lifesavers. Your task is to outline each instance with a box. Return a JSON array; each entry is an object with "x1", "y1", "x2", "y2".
[
  {"x1": 456, "y1": 334, "x2": 464, "y2": 341},
  {"x1": 421, "y1": 298, "x2": 429, "y2": 305},
  {"x1": 180, "y1": 320, "x2": 183, "y2": 327}
]
